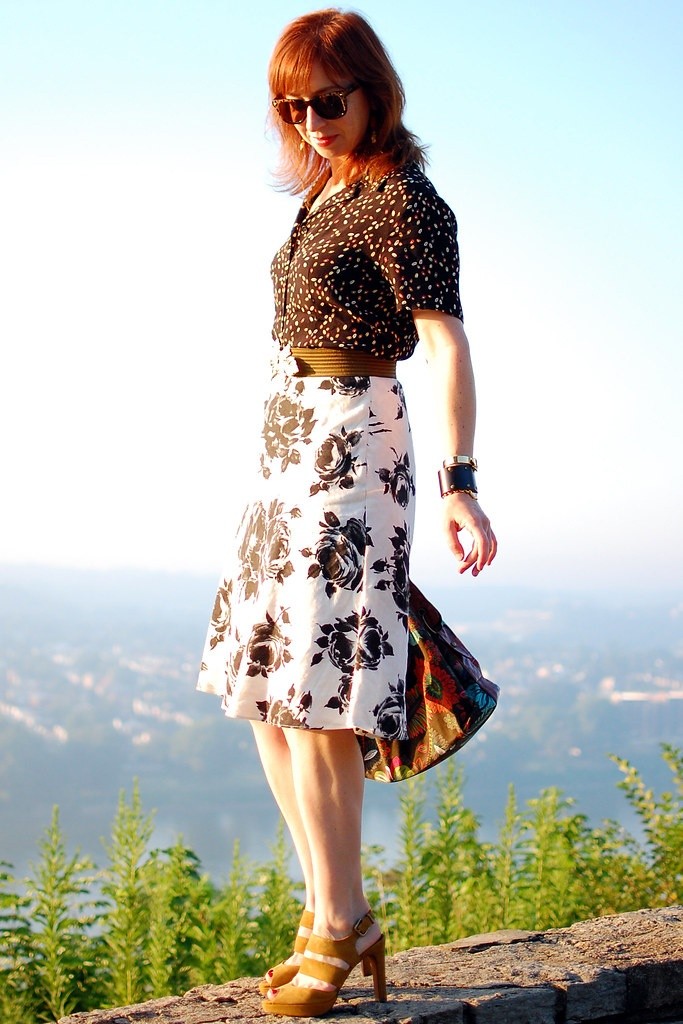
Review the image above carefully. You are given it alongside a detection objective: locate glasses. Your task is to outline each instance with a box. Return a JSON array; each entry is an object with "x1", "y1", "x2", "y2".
[{"x1": 272, "y1": 80, "x2": 363, "y2": 124}]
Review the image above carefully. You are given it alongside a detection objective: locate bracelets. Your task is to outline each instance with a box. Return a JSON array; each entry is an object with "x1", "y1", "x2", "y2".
[
  {"x1": 442, "y1": 455, "x2": 478, "y2": 470},
  {"x1": 438, "y1": 465, "x2": 478, "y2": 500}
]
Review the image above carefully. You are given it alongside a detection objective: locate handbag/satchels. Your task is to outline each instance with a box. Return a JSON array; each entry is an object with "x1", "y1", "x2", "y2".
[{"x1": 355, "y1": 578, "x2": 501, "y2": 784}]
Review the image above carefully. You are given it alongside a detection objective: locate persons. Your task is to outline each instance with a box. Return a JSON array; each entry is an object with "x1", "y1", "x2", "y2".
[{"x1": 197, "y1": 8, "x2": 498, "y2": 1018}]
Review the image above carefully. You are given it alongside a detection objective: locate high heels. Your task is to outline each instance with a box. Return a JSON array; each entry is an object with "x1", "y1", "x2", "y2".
[
  {"x1": 259, "y1": 909, "x2": 373, "y2": 995},
  {"x1": 262, "y1": 909, "x2": 387, "y2": 1017}
]
